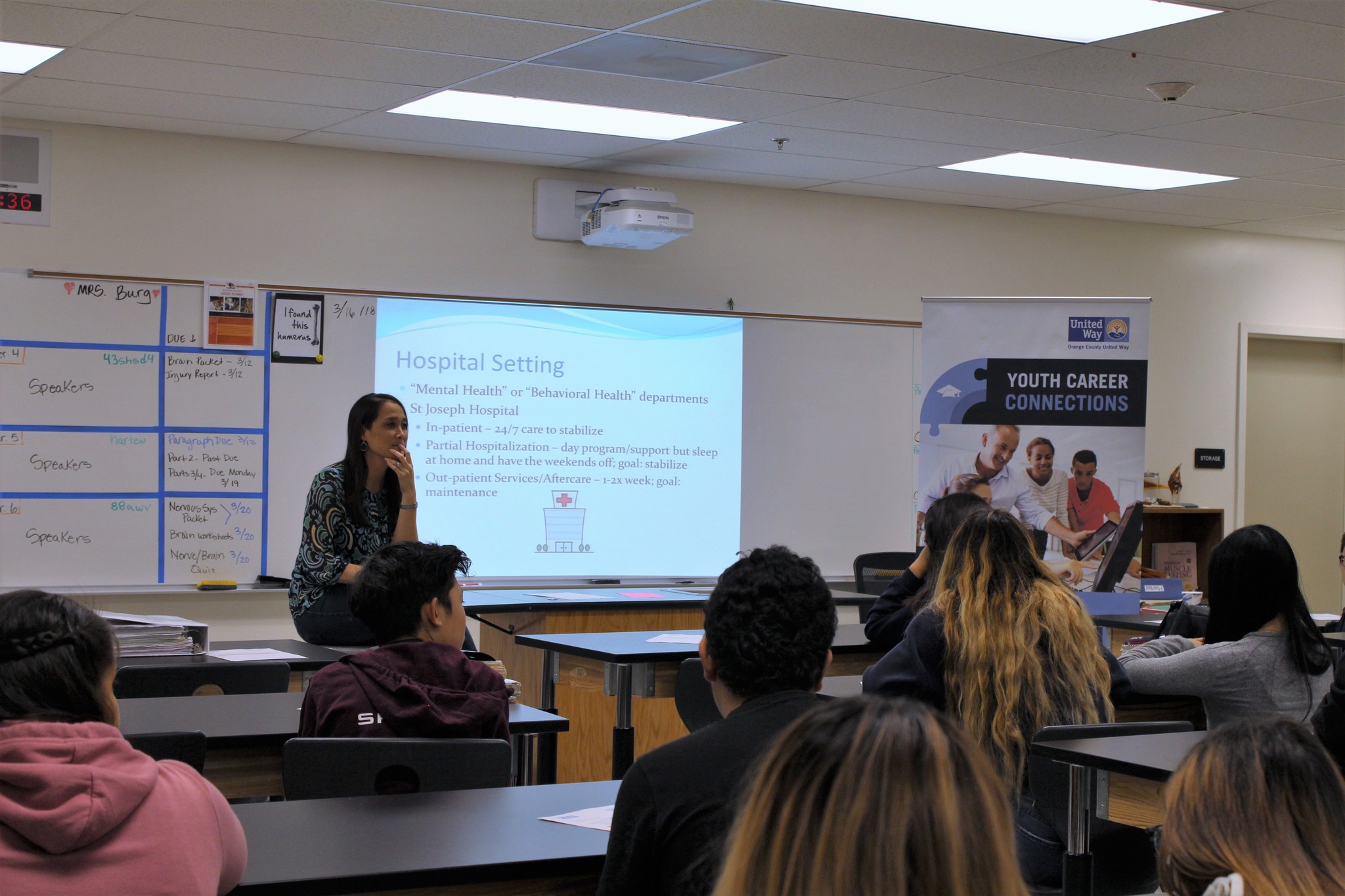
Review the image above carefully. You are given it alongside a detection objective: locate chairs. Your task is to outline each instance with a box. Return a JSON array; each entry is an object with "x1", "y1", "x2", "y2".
[
  {"x1": 851, "y1": 550, "x2": 922, "y2": 624},
  {"x1": 117, "y1": 729, "x2": 213, "y2": 779},
  {"x1": 1012, "y1": 720, "x2": 1198, "y2": 889},
  {"x1": 277, "y1": 739, "x2": 516, "y2": 799},
  {"x1": 107, "y1": 663, "x2": 297, "y2": 697},
  {"x1": 666, "y1": 652, "x2": 730, "y2": 737}
]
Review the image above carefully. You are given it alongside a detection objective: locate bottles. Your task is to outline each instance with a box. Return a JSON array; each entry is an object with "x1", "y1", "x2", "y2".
[{"x1": 1143, "y1": 473, "x2": 1159, "y2": 505}]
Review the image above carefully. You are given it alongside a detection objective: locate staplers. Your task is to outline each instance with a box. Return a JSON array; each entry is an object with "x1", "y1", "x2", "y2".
[{"x1": 252, "y1": 575, "x2": 292, "y2": 589}]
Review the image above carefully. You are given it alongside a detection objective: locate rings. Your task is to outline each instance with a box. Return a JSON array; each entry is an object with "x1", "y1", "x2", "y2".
[{"x1": 395, "y1": 462, "x2": 401, "y2": 468}]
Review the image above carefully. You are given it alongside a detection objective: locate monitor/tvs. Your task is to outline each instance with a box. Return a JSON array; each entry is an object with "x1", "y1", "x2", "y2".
[
  {"x1": 1090, "y1": 500, "x2": 1142, "y2": 592},
  {"x1": 1072, "y1": 519, "x2": 1119, "y2": 562}
]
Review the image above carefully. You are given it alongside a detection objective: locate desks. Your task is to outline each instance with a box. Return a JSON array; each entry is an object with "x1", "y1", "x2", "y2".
[{"x1": 0, "y1": 582, "x2": 1345, "y2": 896}]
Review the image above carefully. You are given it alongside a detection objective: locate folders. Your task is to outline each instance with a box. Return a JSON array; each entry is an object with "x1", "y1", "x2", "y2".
[{"x1": 1075, "y1": 591, "x2": 1141, "y2": 615}]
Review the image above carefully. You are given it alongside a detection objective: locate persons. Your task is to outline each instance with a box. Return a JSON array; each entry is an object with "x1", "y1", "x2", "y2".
[
  {"x1": 865, "y1": 492, "x2": 993, "y2": 647},
  {"x1": 288, "y1": 393, "x2": 478, "y2": 652},
  {"x1": 0, "y1": 589, "x2": 247, "y2": 896},
  {"x1": 298, "y1": 541, "x2": 513, "y2": 744},
  {"x1": 1316, "y1": 534, "x2": 1345, "y2": 634},
  {"x1": 597, "y1": 546, "x2": 838, "y2": 895},
  {"x1": 943, "y1": 474, "x2": 994, "y2": 512},
  {"x1": 916, "y1": 425, "x2": 1121, "y2": 585},
  {"x1": 1313, "y1": 533, "x2": 1344, "y2": 775},
  {"x1": 1144, "y1": 721, "x2": 1345, "y2": 896},
  {"x1": 863, "y1": 492, "x2": 1131, "y2": 889},
  {"x1": 1116, "y1": 526, "x2": 1334, "y2": 731}
]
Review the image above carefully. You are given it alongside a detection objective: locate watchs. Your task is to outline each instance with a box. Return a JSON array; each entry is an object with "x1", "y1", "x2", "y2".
[{"x1": 398, "y1": 502, "x2": 417, "y2": 510}]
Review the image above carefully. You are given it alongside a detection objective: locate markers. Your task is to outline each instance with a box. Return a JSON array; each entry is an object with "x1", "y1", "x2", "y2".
[
  {"x1": 675, "y1": 581, "x2": 694, "y2": 583},
  {"x1": 459, "y1": 583, "x2": 483, "y2": 587}
]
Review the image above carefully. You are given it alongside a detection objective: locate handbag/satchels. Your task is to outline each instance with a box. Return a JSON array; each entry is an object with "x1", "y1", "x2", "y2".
[{"x1": 1150, "y1": 600, "x2": 1210, "y2": 639}]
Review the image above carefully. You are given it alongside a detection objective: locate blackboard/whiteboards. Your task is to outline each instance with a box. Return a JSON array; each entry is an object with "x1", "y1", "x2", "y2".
[{"x1": 0, "y1": 265, "x2": 922, "y2": 593}]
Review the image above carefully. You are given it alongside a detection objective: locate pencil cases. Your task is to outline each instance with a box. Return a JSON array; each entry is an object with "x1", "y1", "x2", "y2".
[{"x1": 1125, "y1": 635, "x2": 1153, "y2": 645}]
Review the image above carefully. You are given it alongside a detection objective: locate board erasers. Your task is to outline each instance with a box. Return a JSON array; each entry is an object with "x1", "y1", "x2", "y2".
[
  {"x1": 198, "y1": 580, "x2": 237, "y2": 591},
  {"x1": 591, "y1": 579, "x2": 621, "y2": 585}
]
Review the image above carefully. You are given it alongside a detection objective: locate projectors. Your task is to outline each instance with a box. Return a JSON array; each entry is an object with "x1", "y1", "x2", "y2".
[{"x1": 580, "y1": 201, "x2": 693, "y2": 251}]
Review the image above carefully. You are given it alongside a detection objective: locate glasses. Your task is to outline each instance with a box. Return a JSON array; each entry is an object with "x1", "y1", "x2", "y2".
[{"x1": 1339, "y1": 555, "x2": 1345, "y2": 566}]
